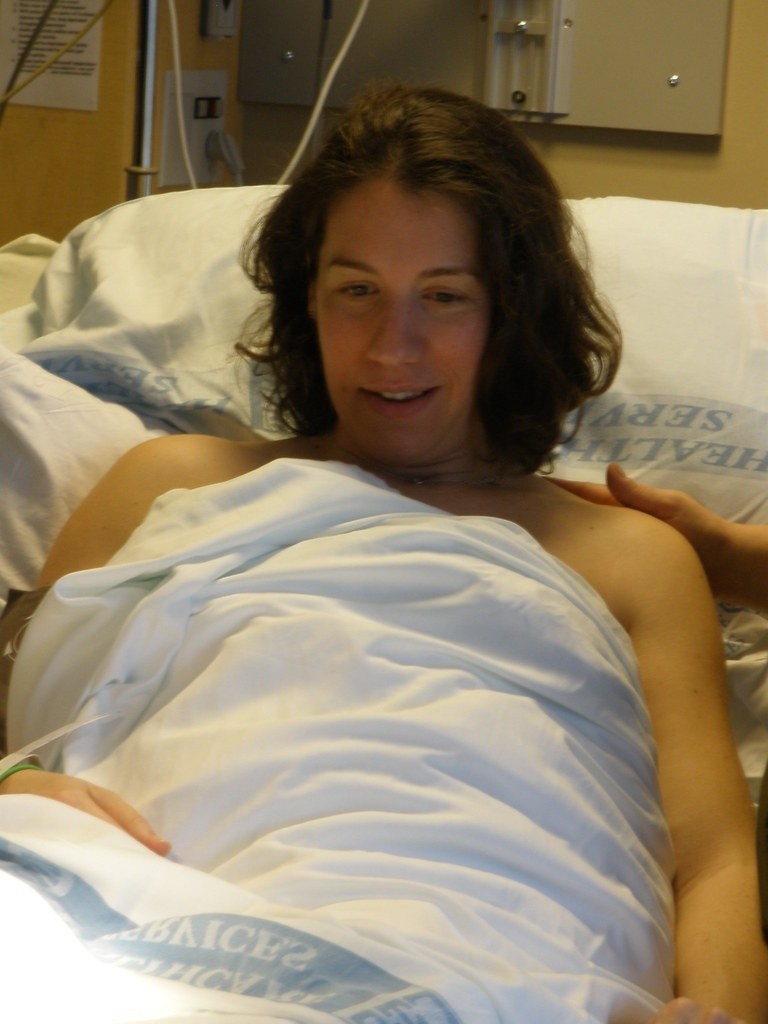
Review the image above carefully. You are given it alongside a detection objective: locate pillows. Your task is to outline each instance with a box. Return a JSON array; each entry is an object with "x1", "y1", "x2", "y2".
[{"x1": 37, "y1": 185, "x2": 768, "y2": 531}]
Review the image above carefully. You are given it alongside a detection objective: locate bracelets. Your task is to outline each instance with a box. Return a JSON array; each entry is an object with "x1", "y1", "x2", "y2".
[{"x1": 0, "y1": 713, "x2": 109, "y2": 785}]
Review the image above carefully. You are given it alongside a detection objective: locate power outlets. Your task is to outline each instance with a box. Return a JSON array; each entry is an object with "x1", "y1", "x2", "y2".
[{"x1": 165, "y1": 68, "x2": 228, "y2": 190}]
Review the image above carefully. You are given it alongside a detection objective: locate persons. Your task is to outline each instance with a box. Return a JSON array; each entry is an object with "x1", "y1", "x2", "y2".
[
  {"x1": 0, "y1": 89, "x2": 767, "y2": 1024},
  {"x1": 543, "y1": 463, "x2": 767, "y2": 620}
]
